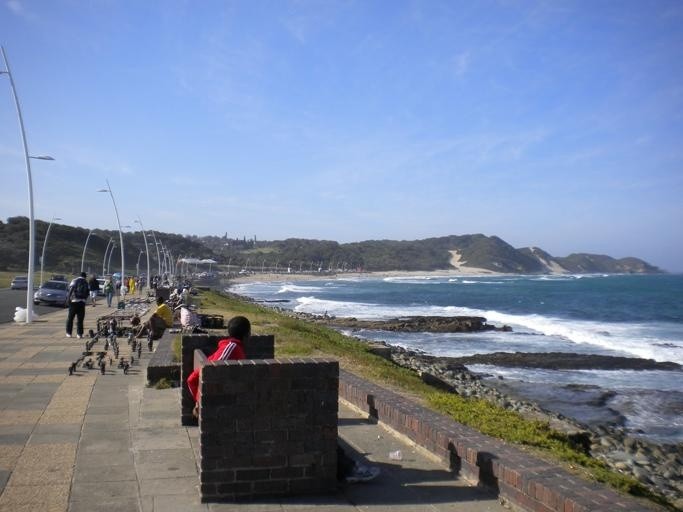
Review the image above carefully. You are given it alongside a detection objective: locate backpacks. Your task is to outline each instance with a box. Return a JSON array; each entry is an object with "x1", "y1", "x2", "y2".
[{"x1": 73, "y1": 277, "x2": 90, "y2": 299}]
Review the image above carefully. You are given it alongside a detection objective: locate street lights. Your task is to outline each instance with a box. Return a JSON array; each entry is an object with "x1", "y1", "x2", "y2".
[
  {"x1": 0, "y1": 46, "x2": 62, "y2": 324},
  {"x1": 81, "y1": 179, "x2": 266, "y2": 297}
]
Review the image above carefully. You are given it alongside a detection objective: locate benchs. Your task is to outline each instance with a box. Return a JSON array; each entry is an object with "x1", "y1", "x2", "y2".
[
  {"x1": 179, "y1": 332, "x2": 339, "y2": 503},
  {"x1": 147, "y1": 327, "x2": 198, "y2": 386}
]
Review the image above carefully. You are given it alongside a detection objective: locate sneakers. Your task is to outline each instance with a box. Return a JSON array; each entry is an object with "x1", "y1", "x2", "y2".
[{"x1": 66, "y1": 333, "x2": 82, "y2": 339}]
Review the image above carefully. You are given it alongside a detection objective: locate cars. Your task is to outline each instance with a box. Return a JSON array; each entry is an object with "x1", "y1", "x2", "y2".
[{"x1": 33, "y1": 274, "x2": 71, "y2": 309}]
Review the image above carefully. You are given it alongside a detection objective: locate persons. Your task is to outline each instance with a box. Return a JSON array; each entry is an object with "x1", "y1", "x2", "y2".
[
  {"x1": 88, "y1": 272, "x2": 196, "y2": 308},
  {"x1": 133, "y1": 298, "x2": 173, "y2": 340},
  {"x1": 64, "y1": 271, "x2": 88, "y2": 339},
  {"x1": 185, "y1": 317, "x2": 381, "y2": 484}
]
